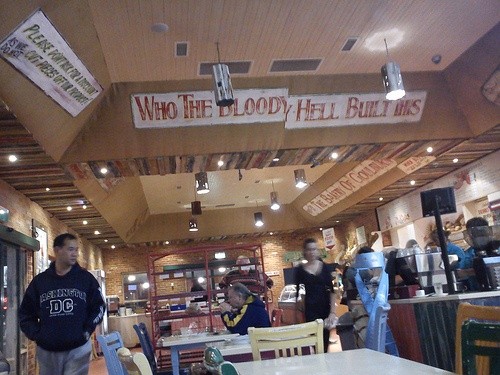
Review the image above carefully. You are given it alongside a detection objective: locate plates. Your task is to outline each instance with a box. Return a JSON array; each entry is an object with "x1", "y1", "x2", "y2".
[
  {"x1": 413, "y1": 295, "x2": 429, "y2": 297},
  {"x1": 432, "y1": 293, "x2": 449, "y2": 296}
]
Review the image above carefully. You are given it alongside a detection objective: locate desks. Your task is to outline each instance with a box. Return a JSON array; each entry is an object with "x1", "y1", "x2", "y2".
[
  {"x1": 231, "y1": 348, "x2": 460, "y2": 375},
  {"x1": 109, "y1": 313, "x2": 152, "y2": 347},
  {"x1": 156, "y1": 331, "x2": 241, "y2": 375}
]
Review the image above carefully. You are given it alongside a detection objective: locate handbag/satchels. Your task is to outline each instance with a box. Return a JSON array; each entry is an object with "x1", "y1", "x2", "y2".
[{"x1": 340, "y1": 297, "x2": 347, "y2": 305}]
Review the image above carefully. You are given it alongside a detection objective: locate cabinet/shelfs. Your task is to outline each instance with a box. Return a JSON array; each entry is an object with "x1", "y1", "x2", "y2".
[
  {"x1": 148, "y1": 243, "x2": 270, "y2": 369},
  {"x1": 389, "y1": 191, "x2": 500, "y2": 248}
]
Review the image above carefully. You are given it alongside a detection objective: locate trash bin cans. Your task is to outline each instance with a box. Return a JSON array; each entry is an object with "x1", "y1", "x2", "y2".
[{"x1": 336, "y1": 308, "x2": 368, "y2": 350}]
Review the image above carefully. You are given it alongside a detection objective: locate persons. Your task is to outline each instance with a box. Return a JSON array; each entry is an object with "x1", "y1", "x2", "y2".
[
  {"x1": 191, "y1": 279, "x2": 208, "y2": 302},
  {"x1": 221, "y1": 283, "x2": 271, "y2": 335},
  {"x1": 20, "y1": 233, "x2": 106, "y2": 375},
  {"x1": 343, "y1": 217, "x2": 500, "y2": 305},
  {"x1": 293, "y1": 238, "x2": 339, "y2": 353},
  {"x1": 219, "y1": 255, "x2": 273, "y2": 296}
]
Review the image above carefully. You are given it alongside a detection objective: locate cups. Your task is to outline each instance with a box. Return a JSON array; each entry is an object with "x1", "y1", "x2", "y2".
[
  {"x1": 434, "y1": 283, "x2": 443, "y2": 294},
  {"x1": 416, "y1": 290, "x2": 425, "y2": 296},
  {"x1": 120, "y1": 307, "x2": 125, "y2": 316},
  {"x1": 126, "y1": 308, "x2": 132, "y2": 316}
]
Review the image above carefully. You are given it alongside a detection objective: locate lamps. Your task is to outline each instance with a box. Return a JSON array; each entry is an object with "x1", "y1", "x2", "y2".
[
  {"x1": 212, "y1": 42, "x2": 235, "y2": 107},
  {"x1": 195, "y1": 165, "x2": 210, "y2": 195},
  {"x1": 191, "y1": 196, "x2": 202, "y2": 215},
  {"x1": 269, "y1": 180, "x2": 280, "y2": 210},
  {"x1": 254, "y1": 200, "x2": 264, "y2": 227},
  {"x1": 294, "y1": 169, "x2": 307, "y2": 189},
  {"x1": 189, "y1": 212, "x2": 198, "y2": 232},
  {"x1": 380, "y1": 37, "x2": 406, "y2": 100}
]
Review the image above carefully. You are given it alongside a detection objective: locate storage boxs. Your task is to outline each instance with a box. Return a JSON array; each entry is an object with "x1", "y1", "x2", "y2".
[{"x1": 388, "y1": 285, "x2": 420, "y2": 299}]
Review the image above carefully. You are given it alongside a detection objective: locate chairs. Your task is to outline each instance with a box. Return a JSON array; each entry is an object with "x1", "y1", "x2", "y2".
[
  {"x1": 133, "y1": 322, "x2": 191, "y2": 375},
  {"x1": 96, "y1": 330, "x2": 129, "y2": 375},
  {"x1": 116, "y1": 348, "x2": 154, "y2": 375},
  {"x1": 454, "y1": 301, "x2": 500, "y2": 375},
  {"x1": 247, "y1": 319, "x2": 324, "y2": 360}
]
